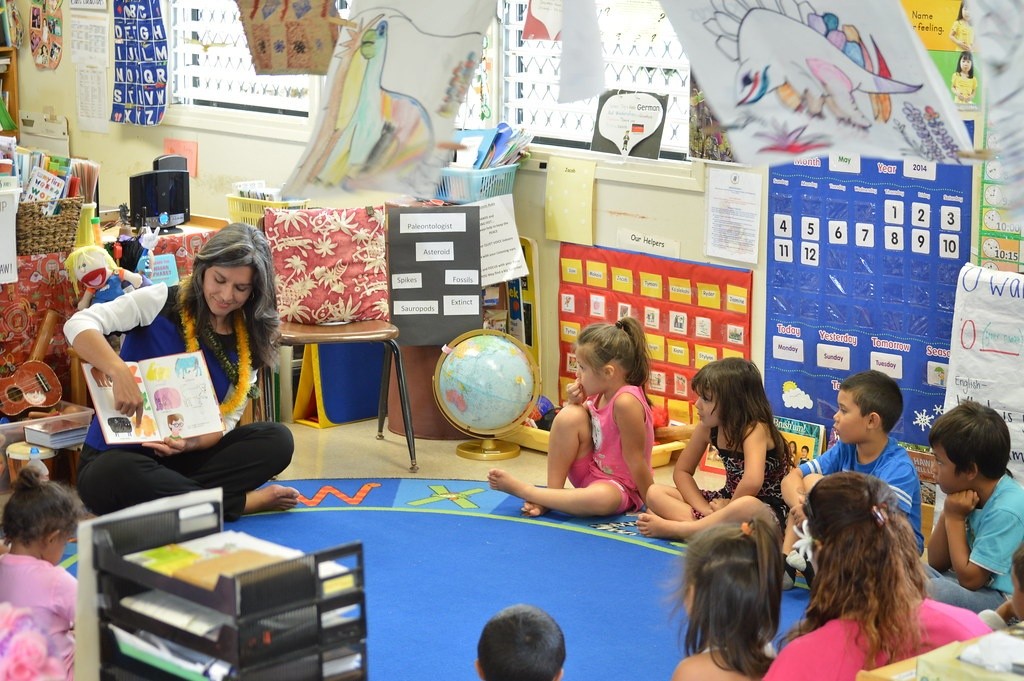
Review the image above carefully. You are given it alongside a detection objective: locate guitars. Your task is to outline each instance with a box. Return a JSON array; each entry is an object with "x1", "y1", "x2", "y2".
[{"x1": 0, "y1": 360, "x2": 63, "y2": 420}]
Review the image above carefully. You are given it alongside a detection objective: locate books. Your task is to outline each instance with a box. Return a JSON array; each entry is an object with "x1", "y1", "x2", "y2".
[
  {"x1": 23, "y1": 420, "x2": 89, "y2": 449},
  {"x1": 0, "y1": 136, "x2": 81, "y2": 284},
  {"x1": 81, "y1": 350, "x2": 226, "y2": 444},
  {"x1": 0, "y1": 0, "x2": 18, "y2": 133},
  {"x1": 108, "y1": 530, "x2": 361, "y2": 681},
  {"x1": 232, "y1": 179, "x2": 281, "y2": 226}
]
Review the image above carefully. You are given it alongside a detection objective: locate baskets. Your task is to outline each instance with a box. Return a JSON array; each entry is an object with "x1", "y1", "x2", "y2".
[
  {"x1": 17, "y1": 194, "x2": 85, "y2": 255},
  {"x1": 434, "y1": 164, "x2": 520, "y2": 204},
  {"x1": 226, "y1": 194, "x2": 310, "y2": 229}
]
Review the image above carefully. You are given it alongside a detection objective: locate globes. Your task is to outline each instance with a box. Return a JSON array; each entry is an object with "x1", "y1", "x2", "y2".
[{"x1": 432, "y1": 329, "x2": 543, "y2": 461}]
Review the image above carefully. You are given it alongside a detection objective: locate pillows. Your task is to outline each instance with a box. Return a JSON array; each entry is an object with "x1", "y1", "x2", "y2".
[{"x1": 264, "y1": 207, "x2": 391, "y2": 324}]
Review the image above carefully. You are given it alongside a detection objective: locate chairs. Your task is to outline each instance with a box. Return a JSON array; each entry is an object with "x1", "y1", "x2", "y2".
[{"x1": 257, "y1": 215, "x2": 418, "y2": 475}]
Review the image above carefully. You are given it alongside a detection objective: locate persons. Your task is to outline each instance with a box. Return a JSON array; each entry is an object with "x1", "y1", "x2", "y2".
[
  {"x1": 488, "y1": 317, "x2": 654, "y2": 517},
  {"x1": 780, "y1": 370, "x2": 925, "y2": 590},
  {"x1": 788, "y1": 441, "x2": 810, "y2": 467},
  {"x1": 635, "y1": 357, "x2": 795, "y2": 543},
  {"x1": 667, "y1": 514, "x2": 782, "y2": 681},
  {"x1": 30, "y1": 0, "x2": 61, "y2": 66},
  {"x1": 474, "y1": 604, "x2": 566, "y2": 681},
  {"x1": 921, "y1": 402, "x2": 1024, "y2": 633},
  {"x1": 761, "y1": 473, "x2": 993, "y2": 681},
  {"x1": 63, "y1": 223, "x2": 300, "y2": 521},
  {"x1": 0, "y1": 467, "x2": 77, "y2": 681}
]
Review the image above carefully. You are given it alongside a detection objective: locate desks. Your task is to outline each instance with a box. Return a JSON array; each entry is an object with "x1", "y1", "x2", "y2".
[{"x1": 0, "y1": 206, "x2": 232, "y2": 405}]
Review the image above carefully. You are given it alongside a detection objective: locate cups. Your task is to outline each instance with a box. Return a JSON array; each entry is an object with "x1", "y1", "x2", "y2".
[{"x1": 72, "y1": 202, "x2": 97, "y2": 245}]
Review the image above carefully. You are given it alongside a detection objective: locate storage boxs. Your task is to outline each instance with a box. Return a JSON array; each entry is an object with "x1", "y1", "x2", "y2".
[{"x1": 0, "y1": 398, "x2": 96, "y2": 490}]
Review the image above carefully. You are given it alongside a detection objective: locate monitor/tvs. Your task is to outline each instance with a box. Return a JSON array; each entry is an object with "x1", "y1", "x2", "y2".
[
  {"x1": 129, "y1": 169, "x2": 191, "y2": 235},
  {"x1": 152, "y1": 154, "x2": 187, "y2": 171}
]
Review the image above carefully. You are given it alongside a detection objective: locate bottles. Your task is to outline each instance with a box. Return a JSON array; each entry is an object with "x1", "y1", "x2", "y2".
[{"x1": 27, "y1": 448, "x2": 49, "y2": 482}]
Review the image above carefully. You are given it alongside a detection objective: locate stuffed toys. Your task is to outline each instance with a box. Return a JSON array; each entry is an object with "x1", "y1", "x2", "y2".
[{"x1": 63, "y1": 245, "x2": 154, "y2": 311}]
